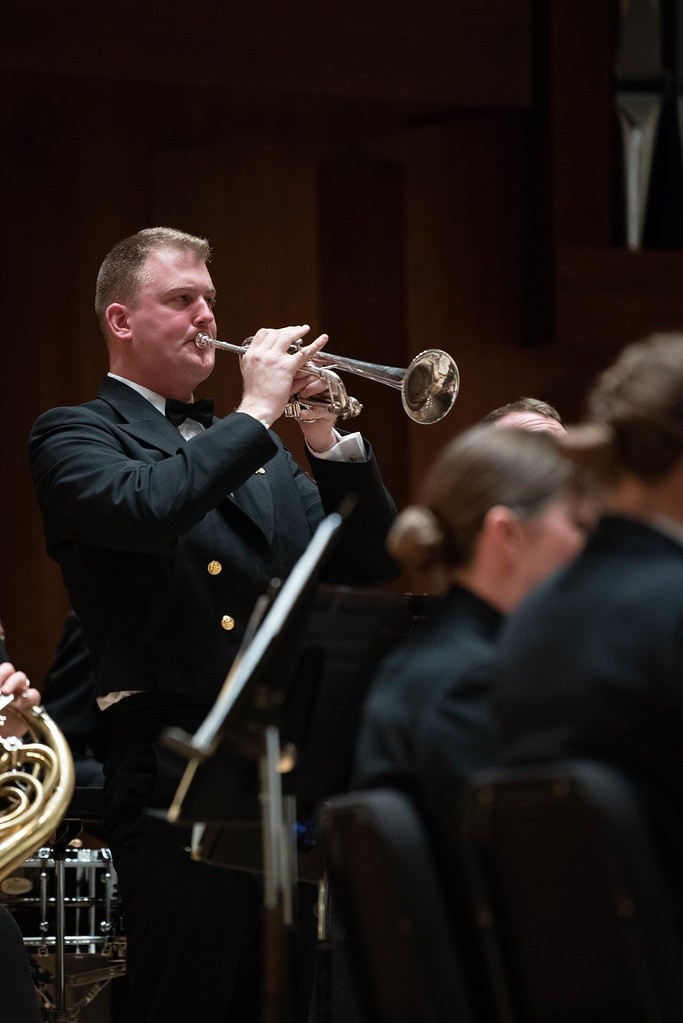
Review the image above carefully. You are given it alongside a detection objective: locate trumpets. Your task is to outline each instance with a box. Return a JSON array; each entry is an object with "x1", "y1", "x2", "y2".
[{"x1": 193, "y1": 330, "x2": 459, "y2": 425}]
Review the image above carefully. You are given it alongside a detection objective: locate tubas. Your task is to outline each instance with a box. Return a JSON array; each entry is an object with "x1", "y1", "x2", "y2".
[{"x1": 0, "y1": 654, "x2": 74, "y2": 891}]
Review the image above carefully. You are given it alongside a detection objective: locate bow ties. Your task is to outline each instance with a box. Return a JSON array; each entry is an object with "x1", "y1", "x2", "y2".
[{"x1": 165, "y1": 396, "x2": 215, "y2": 431}]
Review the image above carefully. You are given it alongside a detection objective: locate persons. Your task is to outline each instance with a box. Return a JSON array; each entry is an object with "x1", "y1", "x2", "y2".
[
  {"x1": 488, "y1": 330, "x2": 683, "y2": 1023},
  {"x1": 0, "y1": 662, "x2": 41, "y2": 1023},
  {"x1": 25, "y1": 225, "x2": 410, "y2": 1022},
  {"x1": 354, "y1": 420, "x2": 587, "y2": 1023}
]
